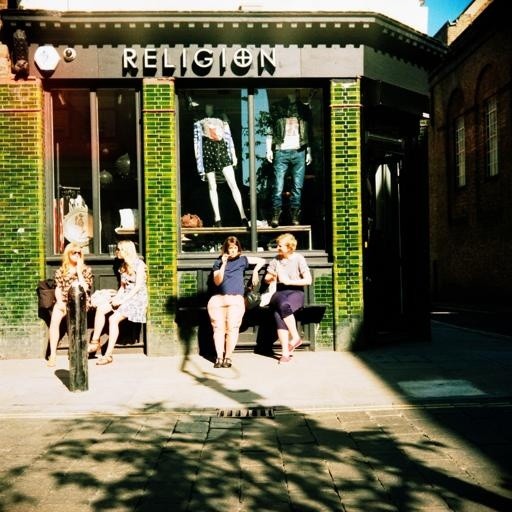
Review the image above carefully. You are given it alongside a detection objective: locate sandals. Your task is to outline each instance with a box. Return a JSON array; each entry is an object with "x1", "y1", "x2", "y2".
[
  {"x1": 47, "y1": 357, "x2": 55, "y2": 367},
  {"x1": 88, "y1": 339, "x2": 101, "y2": 353},
  {"x1": 214, "y1": 357, "x2": 231, "y2": 368},
  {"x1": 97, "y1": 356, "x2": 112, "y2": 365}
]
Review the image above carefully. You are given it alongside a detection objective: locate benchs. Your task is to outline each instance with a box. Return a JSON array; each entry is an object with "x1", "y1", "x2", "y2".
[
  {"x1": 197, "y1": 267, "x2": 326, "y2": 351},
  {"x1": 43, "y1": 257, "x2": 148, "y2": 354}
]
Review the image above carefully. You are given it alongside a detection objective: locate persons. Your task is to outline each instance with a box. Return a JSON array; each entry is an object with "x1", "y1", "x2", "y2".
[
  {"x1": 264, "y1": 232, "x2": 313, "y2": 365},
  {"x1": 266, "y1": 93, "x2": 314, "y2": 227},
  {"x1": 206, "y1": 236, "x2": 266, "y2": 368},
  {"x1": 46, "y1": 241, "x2": 95, "y2": 367},
  {"x1": 193, "y1": 104, "x2": 249, "y2": 227},
  {"x1": 86, "y1": 239, "x2": 149, "y2": 365}
]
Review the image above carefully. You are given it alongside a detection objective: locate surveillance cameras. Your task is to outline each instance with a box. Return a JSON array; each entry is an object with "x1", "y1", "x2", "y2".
[{"x1": 64, "y1": 48, "x2": 76, "y2": 62}]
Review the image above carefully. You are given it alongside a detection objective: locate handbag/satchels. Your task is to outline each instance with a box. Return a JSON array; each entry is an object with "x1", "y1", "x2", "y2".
[
  {"x1": 38, "y1": 279, "x2": 55, "y2": 308},
  {"x1": 259, "y1": 281, "x2": 277, "y2": 307},
  {"x1": 246, "y1": 290, "x2": 260, "y2": 310},
  {"x1": 181, "y1": 212, "x2": 202, "y2": 228}
]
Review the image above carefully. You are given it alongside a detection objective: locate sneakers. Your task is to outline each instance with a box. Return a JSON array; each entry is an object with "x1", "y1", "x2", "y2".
[
  {"x1": 279, "y1": 356, "x2": 291, "y2": 362},
  {"x1": 288, "y1": 337, "x2": 302, "y2": 352}
]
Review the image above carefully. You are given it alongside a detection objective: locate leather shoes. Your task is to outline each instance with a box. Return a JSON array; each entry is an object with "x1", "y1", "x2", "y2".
[
  {"x1": 214, "y1": 220, "x2": 221, "y2": 226},
  {"x1": 241, "y1": 217, "x2": 250, "y2": 226}
]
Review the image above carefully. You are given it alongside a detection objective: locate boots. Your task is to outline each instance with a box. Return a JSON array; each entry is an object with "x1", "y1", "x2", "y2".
[
  {"x1": 271, "y1": 207, "x2": 282, "y2": 227},
  {"x1": 289, "y1": 207, "x2": 301, "y2": 225}
]
